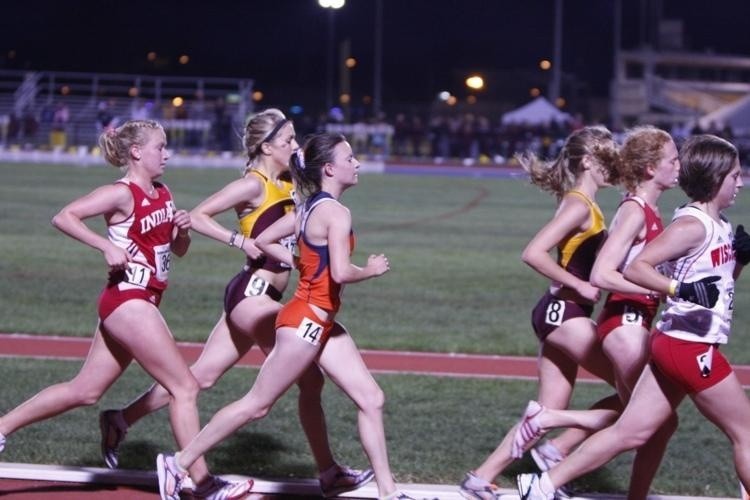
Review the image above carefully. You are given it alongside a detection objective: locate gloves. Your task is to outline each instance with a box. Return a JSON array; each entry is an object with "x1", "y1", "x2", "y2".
[
  {"x1": 669, "y1": 276, "x2": 722, "y2": 309},
  {"x1": 732, "y1": 224, "x2": 750, "y2": 265}
]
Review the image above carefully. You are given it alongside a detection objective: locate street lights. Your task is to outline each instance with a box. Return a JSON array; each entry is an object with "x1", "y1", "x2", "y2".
[{"x1": 318, "y1": 0, "x2": 345, "y2": 107}]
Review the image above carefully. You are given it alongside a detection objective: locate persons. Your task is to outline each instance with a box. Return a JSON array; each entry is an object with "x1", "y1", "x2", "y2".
[
  {"x1": 157, "y1": 133, "x2": 411, "y2": 500},
  {"x1": 0, "y1": 120, "x2": 254, "y2": 500},
  {"x1": 458, "y1": 125, "x2": 624, "y2": 500},
  {"x1": 97, "y1": 108, "x2": 375, "y2": 498},
  {"x1": 0, "y1": 94, "x2": 243, "y2": 152},
  {"x1": 317, "y1": 100, "x2": 750, "y2": 162},
  {"x1": 517, "y1": 135, "x2": 750, "y2": 500},
  {"x1": 513, "y1": 124, "x2": 681, "y2": 500}
]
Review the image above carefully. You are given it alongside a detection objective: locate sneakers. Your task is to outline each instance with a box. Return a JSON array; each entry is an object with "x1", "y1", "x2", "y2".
[
  {"x1": 457, "y1": 470, "x2": 502, "y2": 500},
  {"x1": 155, "y1": 451, "x2": 188, "y2": 500},
  {"x1": 318, "y1": 465, "x2": 375, "y2": 499},
  {"x1": 98, "y1": 409, "x2": 127, "y2": 470},
  {"x1": 378, "y1": 488, "x2": 440, "y2": 500},
  {"x1": 530, "y1": 437, "x2": 575, "y2": 500},
  {"x1": 516, "y1": 473, "x2": 554, "y2": 500},
  {"x1": 191, "y1": 475, "x2": 254, "y2": 500},
  {"x1": 511, "y1": 399, "x2": 546, "y2": 460}
]
